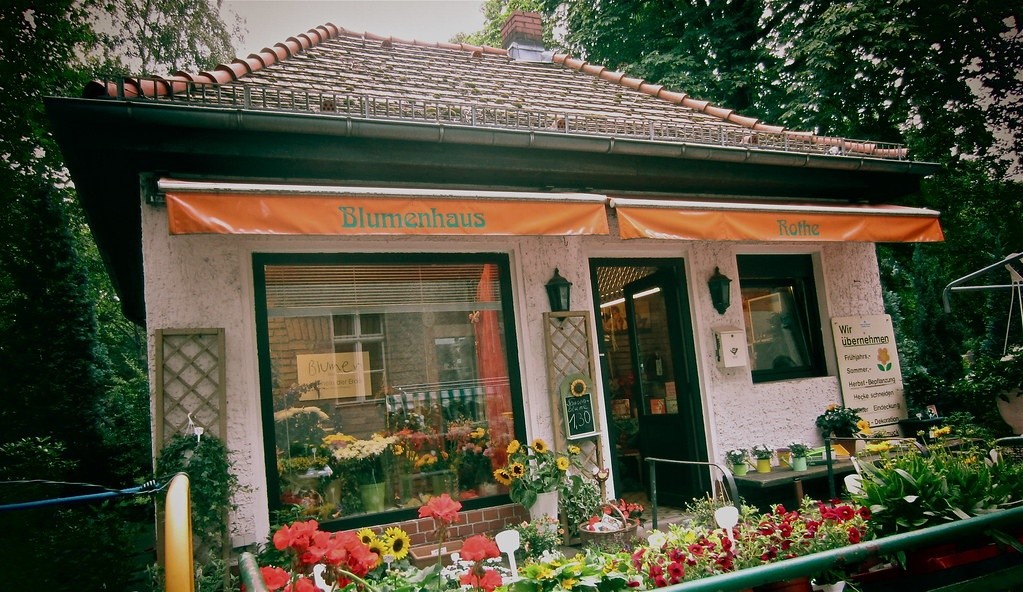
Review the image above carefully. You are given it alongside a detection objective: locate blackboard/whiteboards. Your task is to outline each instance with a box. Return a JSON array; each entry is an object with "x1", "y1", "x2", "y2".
[{"x1": 563, "y1": 393, "x2": 597, "y2": 440}]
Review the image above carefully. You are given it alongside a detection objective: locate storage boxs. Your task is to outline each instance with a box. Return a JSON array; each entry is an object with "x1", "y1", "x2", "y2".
[{"x1": 711, "y1": 325, "x2": 747, "y2": 368}]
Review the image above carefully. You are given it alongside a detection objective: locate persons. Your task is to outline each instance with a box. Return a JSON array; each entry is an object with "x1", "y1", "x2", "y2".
[
  {"x1": 772, "y1": 355, "x2": 797, "y2": 369},
  {"x1": 604, "y1": 305, "x2": 628, "y2": 331}
]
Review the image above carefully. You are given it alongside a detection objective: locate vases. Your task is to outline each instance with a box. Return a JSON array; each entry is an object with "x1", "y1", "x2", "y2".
[
  {"x1": 285, "y1": 465, "x2": 498, "y2": 519},
  {"x1": 745, "y1": 457, "x2": 772, "y2": 473},
  {"x1": 408, "y1": 540, "x2": 465, "y2": 571},
  {"x1": 726, "y1": 462, "x2": 750, "y2": 476},
  {"x1": 897, "y1": 416, "x2": 944, "y2": 445},
  {"x1": 832, "y1": 437, "x2": 858, "y2": 460},
  {"x1": 779, "y1": 455, "x2": 812, "y2": 472},
  {"x1": 528, "y1": 489, "x2": 559, "y2": 541}
]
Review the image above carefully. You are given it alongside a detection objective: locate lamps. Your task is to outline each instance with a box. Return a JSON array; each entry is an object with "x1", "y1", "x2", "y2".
[
  {"x1": 544, "y1": 267, "x2": 573, "y2": 322},
  {"x1": 707, "y1": 266, "x2": 733, "y2": 315}
]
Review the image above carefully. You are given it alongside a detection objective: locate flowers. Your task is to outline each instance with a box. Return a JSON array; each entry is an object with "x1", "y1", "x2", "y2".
[
  {"x1": 787, "y1": 439, "x2": 813, "y2": 457},
  {"x1": 493, "y1": 439, "x2": 581, "y2": 510},
  {"x1": 750, "y1": 444, "x2": 774, "y2": 459},
  {"x1": 814, "y1": 404, "x2": 873, "y2": 437},
  {"x1": 726, "y1": 448, "x2": 748, "y2": 465}
]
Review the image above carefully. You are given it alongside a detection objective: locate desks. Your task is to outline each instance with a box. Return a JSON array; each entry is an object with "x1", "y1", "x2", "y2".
[{"x1": 722, "y1": 436, "x2": 984, "y2": 512}]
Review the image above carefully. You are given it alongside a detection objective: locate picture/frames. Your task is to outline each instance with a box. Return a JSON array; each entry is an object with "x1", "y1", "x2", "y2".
[{"x1": 748, "y1": 292, "x2": 783, "y2": 343}]
